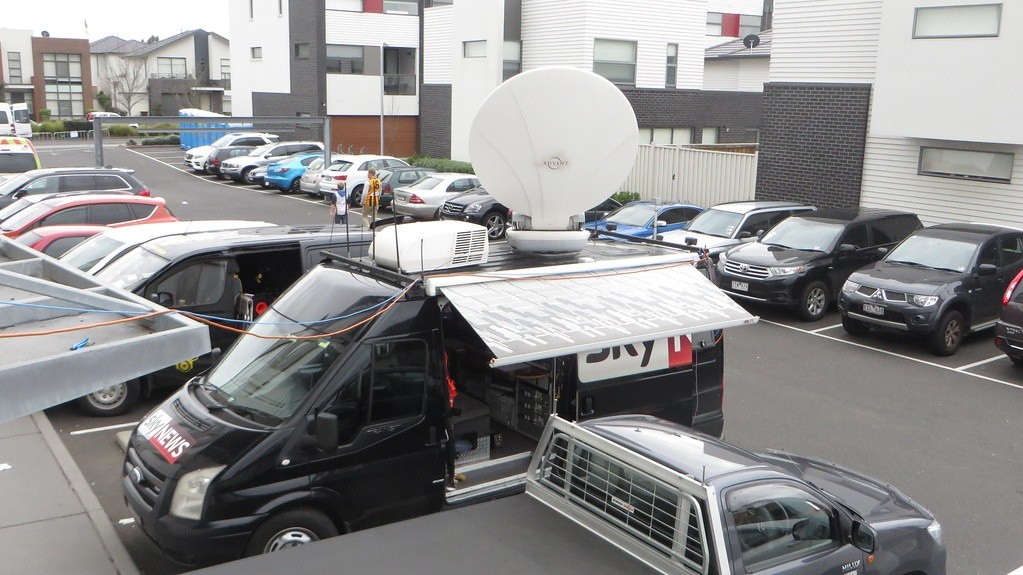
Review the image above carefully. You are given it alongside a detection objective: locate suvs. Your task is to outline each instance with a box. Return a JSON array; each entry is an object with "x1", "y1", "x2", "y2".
[
  {"x1": 715, "y1": 206, "x2": 925, "y2": 322},
  {"x1": 220, "y1": 142, "x2": 324, "y2": 185},
  {"x1": 183, "y1": 134, "x2": 281, "y2": 175},
  {"x1": 835, "y1": 223, "x2": 1023, "y2": 356},
  {"x1": 86, "y1": 112, "x2": 139, "y2": 129},
  {"x1": 640, "y1": 201, "x2": 818, "y2": 281},
  {"x1": 319, "y1": 156, "x2": 412, "y2": 207},
  {"x1": 0, "y1": 167, "x2": 150, "y2": 206}
]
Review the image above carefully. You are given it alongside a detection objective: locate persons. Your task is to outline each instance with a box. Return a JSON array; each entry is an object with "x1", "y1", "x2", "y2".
[
  {"x1": 331, "y1": 183, "x2": 352, "y2": 224},
  {"x1": 360, "y1": 169, "x2": 383, "y2": 227}
]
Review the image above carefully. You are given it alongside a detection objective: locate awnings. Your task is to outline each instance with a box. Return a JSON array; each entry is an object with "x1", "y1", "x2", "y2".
[{"x1": 427, "y1": 255, "x2": 758, "y2": 367}]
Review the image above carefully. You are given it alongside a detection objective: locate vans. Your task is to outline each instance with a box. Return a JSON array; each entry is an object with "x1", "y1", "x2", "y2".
[
  {"x1": 69, "y1": 224, "x2": 382, "y2": 416},
  {"x1": 121, "y1": 232, "x2": 763, "y2": 557},
  {"x1": 0, "y1": 137, "x2": 41, "y2": 184},
  {"x1": 0, "y1": 103, "x2": 32, "y2": 137},
  {"x1": 52, "y1": 220, "x2": 279, "y2": 281}
]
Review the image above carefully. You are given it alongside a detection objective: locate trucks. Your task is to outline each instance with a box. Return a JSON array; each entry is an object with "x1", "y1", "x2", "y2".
[{"x1": 114, "y1": 410, "x2": 948, "y2": 575}]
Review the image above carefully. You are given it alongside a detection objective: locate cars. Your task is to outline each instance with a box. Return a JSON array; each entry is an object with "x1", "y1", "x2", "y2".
[
  {"x1": 204, "y1": 145, "x2": 259, "y2": 181},
  {"x1": 580, "y1": 203, "x2": 705, "y2": 245},
  {"x1": 9, "y1": 226, "x2": 115, "y2": 260},
  {"x1": 391, "y1": 173, "x2": 481, "y2": 220},
  {"x1": 0, "y1": 196, "x2": 178, "y2": 248},
  {"x1": 299, "y1": 155, "x2": 339, "y2": 196},
  {"x1": 370, "y1": 166, "x2": 441, "y2": 210},
  {"x1": 264, "y1": 152, "x2": 337, "y2": 195},
  {"x1": 440, "y1": 183, "x2": 623, "y2": 240},
  {"x1": 247, "y1": 156, "x2": 291, "y2": 188},
  {"x1": 993, "y1": 265, "x2": 1023, "y2": 366}
]
